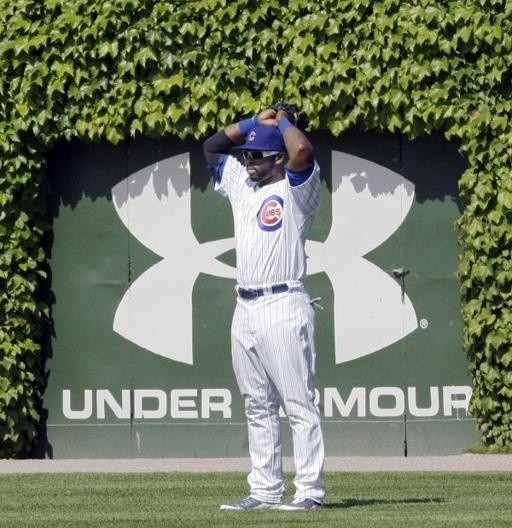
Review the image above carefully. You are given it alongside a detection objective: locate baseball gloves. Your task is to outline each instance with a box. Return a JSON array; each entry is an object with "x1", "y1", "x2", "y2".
[{"x1": 271, "y1": 102, "x2": 309, "y2": 131}]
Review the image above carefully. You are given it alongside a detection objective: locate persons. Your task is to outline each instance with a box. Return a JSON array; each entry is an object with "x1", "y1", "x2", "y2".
[{"x1": 203, "y1": 104, "x2": 327, "y2": 513}]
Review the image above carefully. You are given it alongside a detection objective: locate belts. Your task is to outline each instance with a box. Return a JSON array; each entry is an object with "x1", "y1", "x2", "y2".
[{"x1": 238, "y1": 284, "x2": 287, "y2": 299}]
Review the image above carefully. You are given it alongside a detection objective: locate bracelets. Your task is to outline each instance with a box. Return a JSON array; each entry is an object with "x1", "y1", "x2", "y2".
[
  {"x1": 238, "y1": 114, "x2": 260, "y2": 136},
  {"x1": 277, "y1": 117, "x2": 292, "y2": 135}
]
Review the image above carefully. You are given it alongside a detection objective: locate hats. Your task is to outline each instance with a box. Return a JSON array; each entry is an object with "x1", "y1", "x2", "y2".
[{"x1": 232, "y1": 125, "x2": 285, "y2": 151}]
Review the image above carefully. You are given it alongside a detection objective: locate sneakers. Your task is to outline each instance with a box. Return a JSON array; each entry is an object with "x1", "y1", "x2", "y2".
[
  {"x1": 280, "y1": 498, "x2": 319, "y2": 511},
  {"x1": 220, "y1": 497, "x2": 279, "y2": 512}
]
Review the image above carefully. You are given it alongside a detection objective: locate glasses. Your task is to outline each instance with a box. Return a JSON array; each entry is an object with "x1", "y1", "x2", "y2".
[{"x1": 241, "y1": 150, "x2": 278, "y2": 160}]
